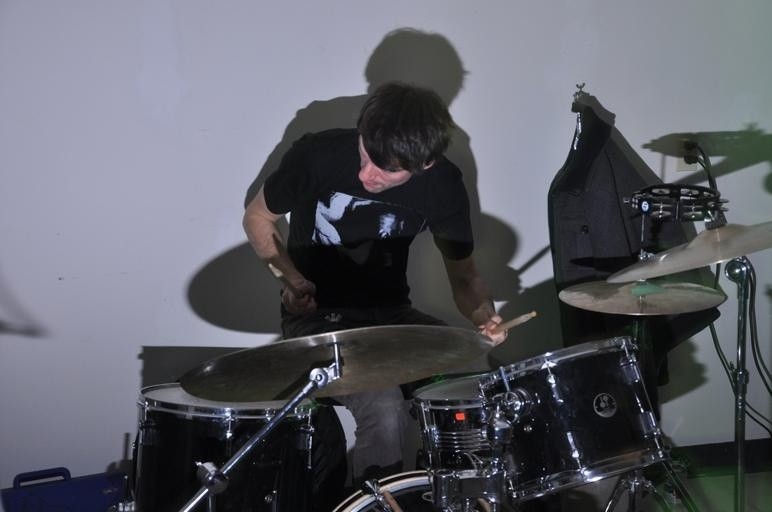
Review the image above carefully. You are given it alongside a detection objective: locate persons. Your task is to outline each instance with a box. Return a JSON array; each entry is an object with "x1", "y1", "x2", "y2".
[{"x1": 241, "y1": 80, "x2": 508, "y2": 487}]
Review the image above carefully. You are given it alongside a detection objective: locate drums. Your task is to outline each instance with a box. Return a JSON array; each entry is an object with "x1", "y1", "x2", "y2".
[
  {"x1": 476, "y1": 338, "x2": 666, "y2": 502},
  {"x1": 331, "y1": 469, "x2": 491, "y2": 511},
  {"x1": 413, "y1": 374, "x2": 499, "y2": 469},
  {"x1": 133, "y1": 383, "x2": 317, "y2": 512}
]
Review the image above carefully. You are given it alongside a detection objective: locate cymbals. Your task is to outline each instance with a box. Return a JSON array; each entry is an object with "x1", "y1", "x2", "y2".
[
  {"x1": 604, "y1": 223, "x2": 772, "y2": 283},
  {"x1": 177, "y1": 324, "x2": 493, "y2": 403},
  {"x1": 558, "y1": 279, "x2": 726, "y2": 313}
]
